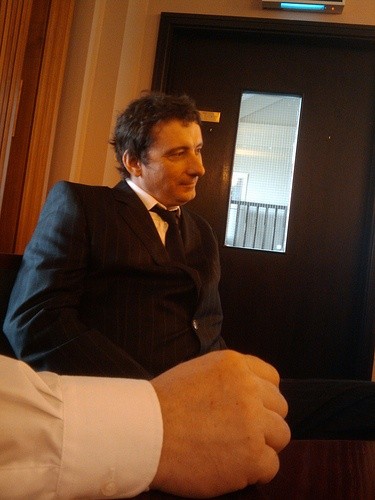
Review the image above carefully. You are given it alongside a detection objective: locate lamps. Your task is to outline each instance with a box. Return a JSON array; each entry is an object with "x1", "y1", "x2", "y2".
[{"x1": 262, "y1": 0, "x2": 345, "y2": 15}]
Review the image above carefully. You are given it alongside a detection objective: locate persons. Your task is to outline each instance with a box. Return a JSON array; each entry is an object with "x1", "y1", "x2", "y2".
[
  {"x1": 2, "y1": 91, "x2": 229, "y2": 382},
  {"x1": 0, "y1": 348, "x2": 291, "y2": 500}
]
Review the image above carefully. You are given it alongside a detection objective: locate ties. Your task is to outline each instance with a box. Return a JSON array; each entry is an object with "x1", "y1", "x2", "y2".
[{"x1": 150, "y1": 204, "x2": 186, "y2": 260}]
